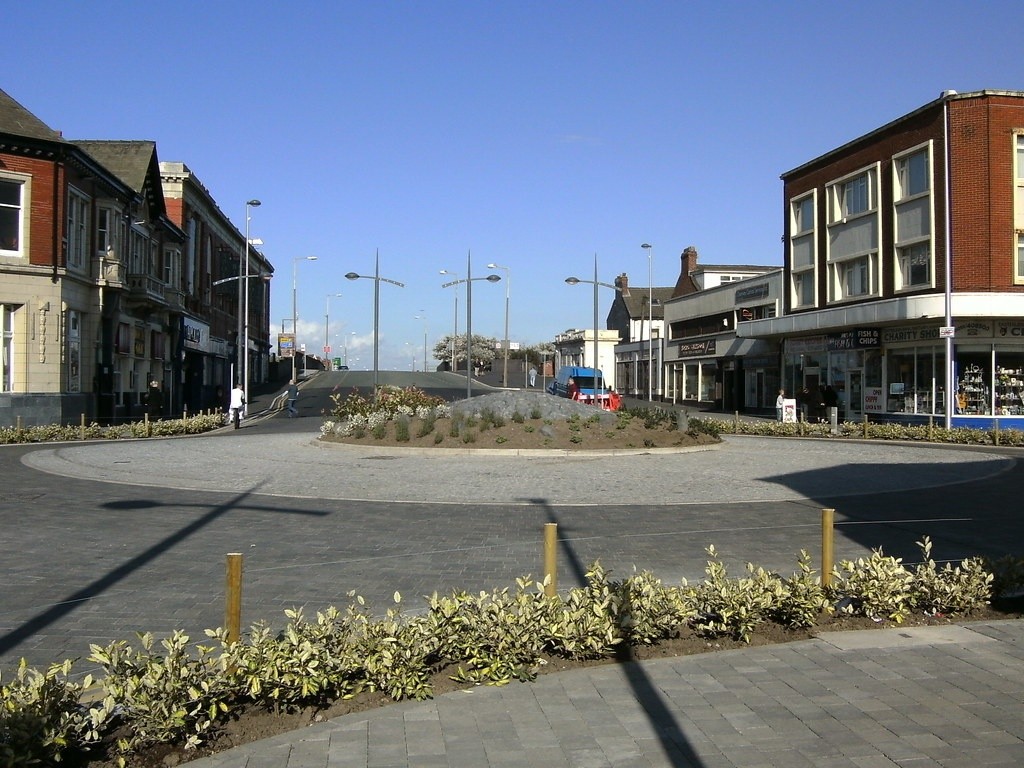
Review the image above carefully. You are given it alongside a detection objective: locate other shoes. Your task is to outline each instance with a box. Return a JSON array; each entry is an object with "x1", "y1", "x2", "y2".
[
  {"x1": 295, "y1": 411, "x2": 299, "y2": 417},
  {"x1": 288, "y1": 415, "x2": 292, "y2": 418},
  {"x1": 239, "y1": 416, "x2": 244, "y2": 420}
]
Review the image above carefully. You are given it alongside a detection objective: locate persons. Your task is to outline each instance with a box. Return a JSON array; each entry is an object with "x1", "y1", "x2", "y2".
[
  {"x1": 282, "y1": 380, "x2": 300, "y2": 418},
  {"x1": 568, "y1": 378, "x2": 578, "y2": 401},
  {"x1": 229, "y1": 384, "x2": 244, "y2": 429},
  {"x1": 473, "y1": 358, "x2": 480, "y2": 376},
  {"x1": 795, "y1": 385, "x2": 835, "y2": 422},
  {"x1": 529, "y1": 367, "x2": 537, "y2": 387},
  {"x1": 147, "y1": 381, "x2": 162, "y2": 420},
  {"x1": 776, "y1": 390, "x2": 785, "y2": 424}
]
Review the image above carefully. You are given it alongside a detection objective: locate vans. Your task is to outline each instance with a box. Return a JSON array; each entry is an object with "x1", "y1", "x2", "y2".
[{"x1": 556, "y1": 365, "x2": 609, "y2": 407}]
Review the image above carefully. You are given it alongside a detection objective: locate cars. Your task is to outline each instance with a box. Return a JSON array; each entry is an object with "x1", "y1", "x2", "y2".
[{"x1": 545, "y1": 378, "x2": 557, "y2": 395}]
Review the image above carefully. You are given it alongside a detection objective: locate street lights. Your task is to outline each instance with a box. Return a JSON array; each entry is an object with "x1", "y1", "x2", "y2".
[
  {"x1": 243, "y1": 198, "x2": 265, "y2": 420},
  {"x1": 441, "y1": 248, "x2": 503, "y2": 400},
  {"x1": 344, "y1": 246, "x2": 405, "y2": 400},
  {"x1": 291, "y1": 255, "x2": 318, "y2": 380},
  {"x1": 214, "y1": 247, "x2": 274, "y2": 385},
  {"x1": 938, "y1": 85, "x2": 960, "y2": 433},
  {"x1": 404, "y1": 341, "x2": 415, "y2": 372},
  {"x1": 642, "y1": 242, "x2": 652, "y2": 402},
  {"x1": 414, "y1": 315, "x2": 427, "y2": 372},
  {"x1": 323, "y1": 293, "x2": 343, "y2": 372},
  {"x1": 565, "y1": 252, "x2": 622, "y2": 410},
  {"x1": 485, "y1": 263, "x2": 510, "y2": 389},
  {"x1": 438, "y1": 268, "x2": 459, "y2": 373}
]
